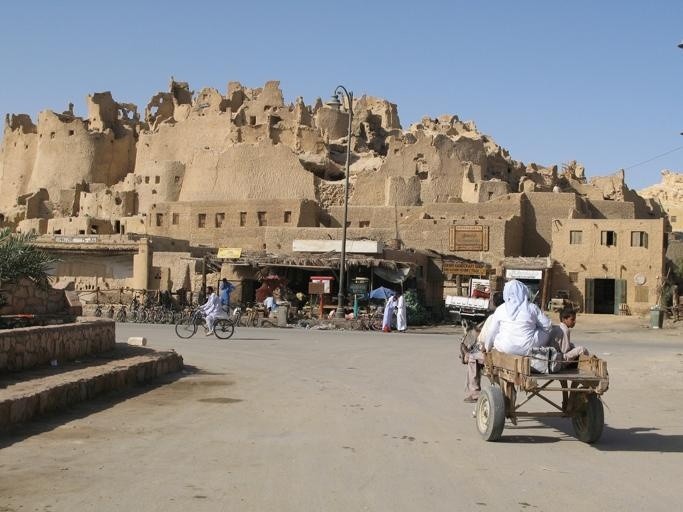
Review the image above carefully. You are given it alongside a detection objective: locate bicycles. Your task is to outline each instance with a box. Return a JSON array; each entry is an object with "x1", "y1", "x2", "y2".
[{"x1": 93, "y1": 293, "x2": 259, "y2": 339}]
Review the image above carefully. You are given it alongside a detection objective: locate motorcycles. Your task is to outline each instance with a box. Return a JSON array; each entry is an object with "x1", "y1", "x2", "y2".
[{"x1": 550, "y1": 289, "x2": 570, "y2": 313}]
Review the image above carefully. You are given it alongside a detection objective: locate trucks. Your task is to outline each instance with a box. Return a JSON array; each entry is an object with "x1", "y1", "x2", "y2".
[{"x1": 445, "y1": 278, "x2": 493, "y2": 328}]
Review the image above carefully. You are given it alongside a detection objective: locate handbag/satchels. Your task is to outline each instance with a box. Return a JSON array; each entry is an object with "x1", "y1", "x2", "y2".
[{"x1": 528, "y1": 346, "x2": 562, "y2": 373}]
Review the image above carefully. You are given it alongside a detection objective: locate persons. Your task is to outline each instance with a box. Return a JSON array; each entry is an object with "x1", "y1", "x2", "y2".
[
  {"x1": 257, "y1": 282, "x2": 309, "y2": 311},
  {"x1": 197, "y1": 286, "x2": 223, "y2": 336},
  {"x1": 382, "y1": 296, "x2": 395, "y2": 332},
  {"x1": 328, "y1": 309, "x2": 360, "y2": 320},
  {"x1": 220, "y1": 278, "x2": 234, "y2": 306},
  {"x1": 462, "y1": 291, "x2": 505, "y2": 402},
  {"x1": 479, "y1": 279, "x2": 553, "y2": 354},
  {"x1": 553, "y1": 183, "x2": 561, "y2": 193},
  {"x1": 472, "y1": 284, "x2": 489, "y2": 298},
  {"x1": 396, "y1": 295, "x2": 408, "y2": 333},
  {"x1": 550, "y1": 307, "x2": 589, "y2": 364}
]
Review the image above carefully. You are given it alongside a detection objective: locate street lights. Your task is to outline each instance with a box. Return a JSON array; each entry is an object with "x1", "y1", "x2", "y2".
[{"x1": 325, "y1": 85, "x2": 353, "y2": 309}]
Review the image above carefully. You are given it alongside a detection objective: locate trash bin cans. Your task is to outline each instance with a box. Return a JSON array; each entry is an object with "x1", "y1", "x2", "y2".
[{"x1": 650, "y1": 309, "x2": 664, "y2": 329}]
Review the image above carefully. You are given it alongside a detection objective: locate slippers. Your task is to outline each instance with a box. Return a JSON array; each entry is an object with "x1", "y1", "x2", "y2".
[{"x1": 463, "y1": 396, "x2": 478, "y2": 403}]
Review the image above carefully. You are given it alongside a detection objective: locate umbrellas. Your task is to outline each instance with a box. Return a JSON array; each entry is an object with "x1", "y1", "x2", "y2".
[{"x1": 366, "y1": 286, "x2": 397, "y2": 299}]
